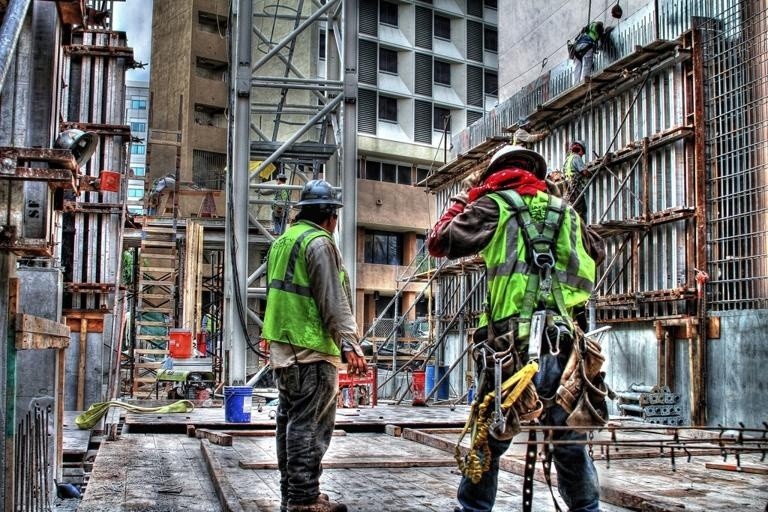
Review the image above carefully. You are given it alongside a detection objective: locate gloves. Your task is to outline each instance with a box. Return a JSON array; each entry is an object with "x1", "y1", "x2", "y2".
[
  {"x1": 542, "y1": 129, "x2": 550, "y2": 137},
  {"x1": 448, "y1": 168, "x2": 484, "y2": 204}
]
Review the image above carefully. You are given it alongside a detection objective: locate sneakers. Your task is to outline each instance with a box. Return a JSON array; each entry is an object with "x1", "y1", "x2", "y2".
[
  {"x1": 279, "y1": 492, "x2": 330, "y2": 512},
  {"x1": 287, "y1": 496, "x2": 348, "y2": 512}
]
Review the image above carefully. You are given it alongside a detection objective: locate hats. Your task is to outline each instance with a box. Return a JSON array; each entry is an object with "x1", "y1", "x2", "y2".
[
  {"x1": 573, "y1": 140, "x2": 587, "y2": 154},
  {"x1": 480, "y1": 144, "x2": 548, "y2": 182},
  {"x1": 518, "y1": 117, "x2": 530, "y2": 127}
]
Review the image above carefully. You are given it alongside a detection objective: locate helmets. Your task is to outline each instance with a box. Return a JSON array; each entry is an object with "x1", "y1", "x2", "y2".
[
  {"x1": 55, "y1": 128, "x2": 98, "y2": 170},
  {"x1": 295, "y1": 179, "x2": 344, "y2": 211},
  {"x1": 276, "y1": 173, "x2": 287, "y2": 180}
]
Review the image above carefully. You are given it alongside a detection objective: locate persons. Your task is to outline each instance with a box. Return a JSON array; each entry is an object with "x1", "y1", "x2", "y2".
[
  {"x1": 201, "y1": 305, "x2": 221, "y2": 356},
  {"x1": 260, "y1": 179, "x2": 369, "y2": 512},
  {"x1": 426, "y1": 145, "x2": 609, "y2": 512},
  {"x1": 569, "y1": 20, "x2": 603, "y2": 87},
  {"x1": 254, "y1": 173, "x2": 289, "y2": 235},
  {"x1": 512, "y1": 116, "x2": 551, "y2": 149},
  {"x1": 561, "y1": 140, "x2": 604, "y2": 224}
]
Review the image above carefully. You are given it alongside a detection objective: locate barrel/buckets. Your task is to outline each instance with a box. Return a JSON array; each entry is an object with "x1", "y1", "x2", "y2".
[
  {"x1": 223, "y1": 385, "x2": 254, "y2": 424},
  {"x1": 169, "y1": 328, "x2": 193, "y2": 359},
  {"x1": 424, "y1": 364, "x2": 451, "y2": 405},
  {"x1": 341, "y1": 387, "x2": 358, "y2": 409},
  {"x1": 196, "y1": 330, "x2": 209, "y2": 359},
  {"x1": 411, "y1": 370, "x2": 426, "y2": 407}
]
